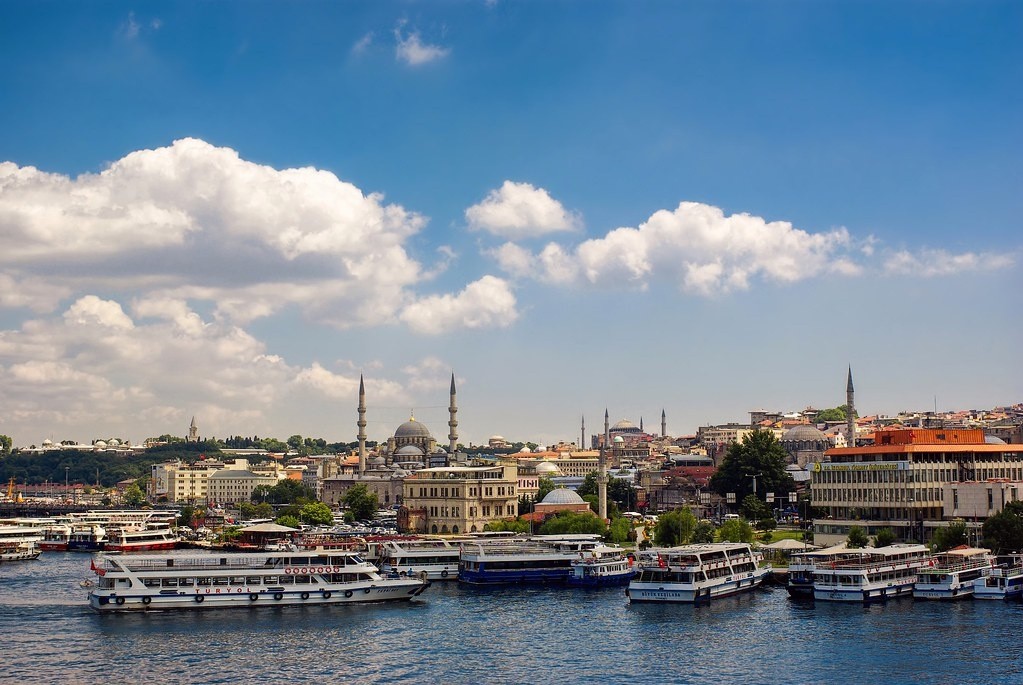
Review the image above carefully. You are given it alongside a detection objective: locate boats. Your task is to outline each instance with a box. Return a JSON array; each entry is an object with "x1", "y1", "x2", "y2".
[
  {"x1": 787, "y1": 540, "x2": 1023, "y2": 603},
  {"x1": 0, "y1": 506, "x2": 184, "y2": 563},
  {"x1": 624, "y1": 541, "x2": 773, "y2": 604},
  {"x1": 568, "y1": 545, "x2": 639, "y2": 589},
  {"x1": 263, "y1": 533, "x2": 606, "y2": 589},
  {"x1": 78, "y1": 547, "x2": 432, "y2": 614}
]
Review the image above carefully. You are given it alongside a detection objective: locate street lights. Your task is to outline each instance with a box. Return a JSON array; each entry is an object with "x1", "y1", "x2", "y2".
[
  {"x1": 65, "y1": 467, "x2": 70, "y2": 499},
  {"x1": 803, "y1": 499, "x2": 809, "y2": 552},
  {"x1": 616, "y1": 501, "x2": 623, "y2": 520},
  {"x1": 909, "y1": 498, "x2": 913, "y2": 541},
  {"x1": 624, "y1": 488, "x2": 630, "y2": 512}
]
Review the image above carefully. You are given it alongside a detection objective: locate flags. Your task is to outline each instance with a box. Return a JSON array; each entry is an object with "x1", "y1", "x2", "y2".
[
  {"x1": 91, "y1": 559, "x2": 106, "y2": 576},
  {"x1": 658, "y1": 553, "x2": 665, "y2": 568}
]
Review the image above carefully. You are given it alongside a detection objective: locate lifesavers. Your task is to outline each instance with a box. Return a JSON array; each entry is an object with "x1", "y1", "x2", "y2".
[
  {"x1": 700, "y1": 554, "x2": 763, "y2": 570},
  {"x1": 637, "y1": 563, "x2": 644, "y2": 569},
  {"x1": 587, "y1": 560, "x2": 591, "y2": 566},
  {"x1": 400, "y1": 570, "x2": 407, "y2": 576},
  {"x1": 974, "y1": 561, "x2": 978, "y2": 567},
  {"x1": 9, "y1": 552, "x2": 43, "y2": 560},
  {"x1": 574, "y1": 560, "x2": 579, "y2": 565},
  {"x1": 116, "y1": 588, "x2": 370, "y2": 606},
  {"x1": 620, "y1": 554, "x2": 624, "y2": 561},
  {"x1": 441, "y1": 570, "x2": 448, "y2": 577},
  {"x1": 680, "y1": 564, "x2": 687, "y2": 570},
  {"x1": 990, "y1": 559, "x2": 994, "y2": 564},
  {"x1": 951, "y1": 566, "x2": 954, "y2": 572},
  {"x1": 863, "y1": 583, "x2": 901, "y2": 598},
  {"x1": 867, "y1": 558, "x2": 939, "y2": 573},
  {"x1": 963, "y1": 563, "x2": 967, "y2": 570},
  {"x1": 284, "y1": 566, "x2": 341, "y2": 575}
]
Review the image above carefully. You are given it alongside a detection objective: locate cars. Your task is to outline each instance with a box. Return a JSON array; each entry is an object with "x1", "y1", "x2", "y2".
[{"x1": 297, "y1": 510, "x2": 398, "y2": 533}]
